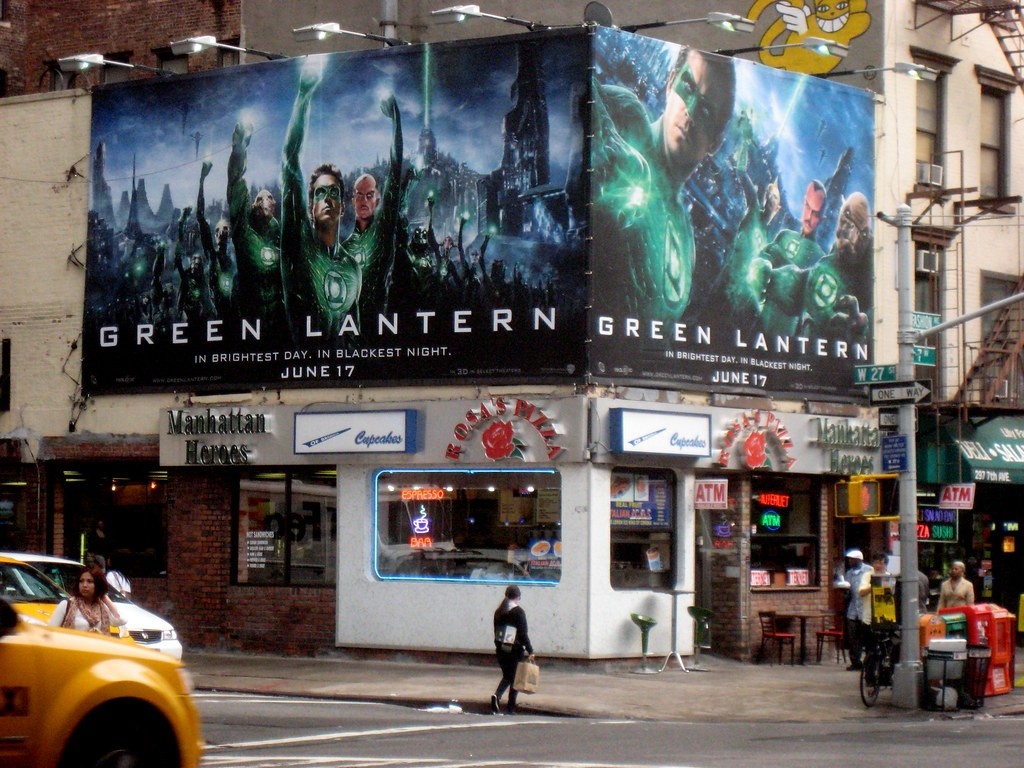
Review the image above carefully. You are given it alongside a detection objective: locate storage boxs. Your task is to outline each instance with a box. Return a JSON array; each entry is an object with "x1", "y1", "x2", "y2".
[
  {"x1": 786, "y1": 569, "x2": 809, "y2": 586},
  {"x1": 750, "y1": 570, "x2": 772, "y2": 585},
  {"x1": 772, "y1": 572, "x2": 786, "y2": 588}
]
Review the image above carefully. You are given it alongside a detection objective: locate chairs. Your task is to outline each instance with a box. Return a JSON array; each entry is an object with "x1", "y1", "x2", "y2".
[
  {"x1": 815, "y1": 607, "x2": 849, "y2": 665},
  {"x1": 756, "y1": 610, "x2": 797, "y2": 668}
]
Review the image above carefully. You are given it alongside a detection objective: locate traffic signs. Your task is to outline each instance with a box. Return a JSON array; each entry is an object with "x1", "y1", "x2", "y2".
[
  {"x1": 868, "y1": 377, "x2": 936, "y2": 409},
  {"x1": 877, "y1": 407, "x2": 919, "y2": 433}
]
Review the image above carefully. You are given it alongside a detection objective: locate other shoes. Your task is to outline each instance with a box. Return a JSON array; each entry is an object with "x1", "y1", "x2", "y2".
[
  {"x1": 504, "y1": 710, "x2": 517, "y2": 716},
  {"x1": 492, "y1": 694, "x2": 499, "y2": 715},
  {"x1": 847, "y1": 661, "x2": 862, "y2": 671}
]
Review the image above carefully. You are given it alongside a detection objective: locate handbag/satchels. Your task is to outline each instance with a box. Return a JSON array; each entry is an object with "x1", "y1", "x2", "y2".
[{"x1": 513, "y1": 658, "x2": 539, "y2": 694}]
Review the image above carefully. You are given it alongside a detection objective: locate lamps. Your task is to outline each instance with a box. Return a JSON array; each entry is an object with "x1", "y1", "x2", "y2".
[
  {"x1": 432, "y1": 3, "x2": 547, "y2": 36},
  {"x1": 622, "y1": 8, "x2": 759, "y2": 38},
  {"x1": 717, "y1": 32, "x2": 854, "y2": 61},
  {"x1": 290, "y1": 18, "x2": 412, "y2": 50},
  {"x1": 817, "y1": 57, "x2": 946, "y2": 86},
  {"x1": 55, "y1": 49, "x2": 171, "y2": 82},
  {"x1": 167, "y1": 31, "x2": 285, "y2": 63}
]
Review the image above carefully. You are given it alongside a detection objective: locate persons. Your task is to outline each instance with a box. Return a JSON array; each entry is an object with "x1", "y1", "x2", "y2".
[
  {"x1": 47, "y1": 565, "x2": 127, "y2": 634},
  {"x1": 937, "y1": 561, "x2": 974, "y2": 612},
  {"x1": 590, "y1": 46, "x2": 737, "y2": 349},
  {"x1": 857, "y1": 553, "x2": 893, "y2": 686},
  {"x1": 703, "y1": 165, "x2": 827, "y2": 342},
  {"x1": 842, "y1": 551, "x2": 873, "y2": 671},
  {"x1": 105, "y1": 553, "x2": 131, "y2": 600},
  {"x1": 491, "y1": 585, "x2": 535, "y2": 714},
  {"x1": 279, "y1": 55, "x2": 403, "y2": 338}
]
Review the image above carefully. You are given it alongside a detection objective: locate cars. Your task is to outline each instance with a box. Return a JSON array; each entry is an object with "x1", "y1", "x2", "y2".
[
  {"x1": 0, "y1": 557, "x2": 130, "y2": 644},
  {"x1": 0, "y1": 596, "x2": 205, "y2": 768},
  {"x1": 0, "y1": 552, "x2": 182, "y2": 660},
  {"x1": 418, "y1": 542, "x2": 562, "y2": 583}
]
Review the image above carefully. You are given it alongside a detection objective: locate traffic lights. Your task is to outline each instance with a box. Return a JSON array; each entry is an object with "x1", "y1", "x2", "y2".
[
  {"x1": 849, "y1": 480, "x2": 881, "y2": 518},
  {"x1": 834, "y1": 480, "x2": 851, "y2": 517}
]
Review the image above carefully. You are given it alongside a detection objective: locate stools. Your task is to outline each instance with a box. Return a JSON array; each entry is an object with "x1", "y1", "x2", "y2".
[
  {"x1": 628, "y1": 611, "x2": 660, "y2": 676},
  {"x1": 684, "y1": 604, "x2": 718, "y2": 674}
]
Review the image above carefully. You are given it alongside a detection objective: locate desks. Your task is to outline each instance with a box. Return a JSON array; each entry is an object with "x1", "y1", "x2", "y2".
[
  {"x1": 653, "y1": 589, "x2": 699, "y2": 675},
  {"x1": 776, "y1": 610, "x2": 838, "y2": 667}
]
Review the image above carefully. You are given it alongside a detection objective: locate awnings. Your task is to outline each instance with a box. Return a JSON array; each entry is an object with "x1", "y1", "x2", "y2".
[{"x1": 946, "y1": 416, "x2": 1024, "y2": 484}]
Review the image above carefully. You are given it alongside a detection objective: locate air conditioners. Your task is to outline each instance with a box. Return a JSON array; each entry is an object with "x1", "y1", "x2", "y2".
[
  {"x1": 916, "y1": 247, "x2": 940, "y2": 275},
  {"x1": 987, "y1": 377, "x2": 1009, "y2": 400},
  {"x1": 918, "y1": 161, "x2": 943, "y2": 187}
]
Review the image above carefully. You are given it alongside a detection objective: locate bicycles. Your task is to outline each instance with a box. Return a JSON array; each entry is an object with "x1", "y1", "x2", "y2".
[{"x1": 859, "y1": 620, "x2": 904, "y2": 709}]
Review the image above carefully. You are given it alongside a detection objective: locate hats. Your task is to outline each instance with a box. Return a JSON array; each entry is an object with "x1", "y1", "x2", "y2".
[{"x1": 847, "y1": 551, "x2": 863, "y2": 560}]
